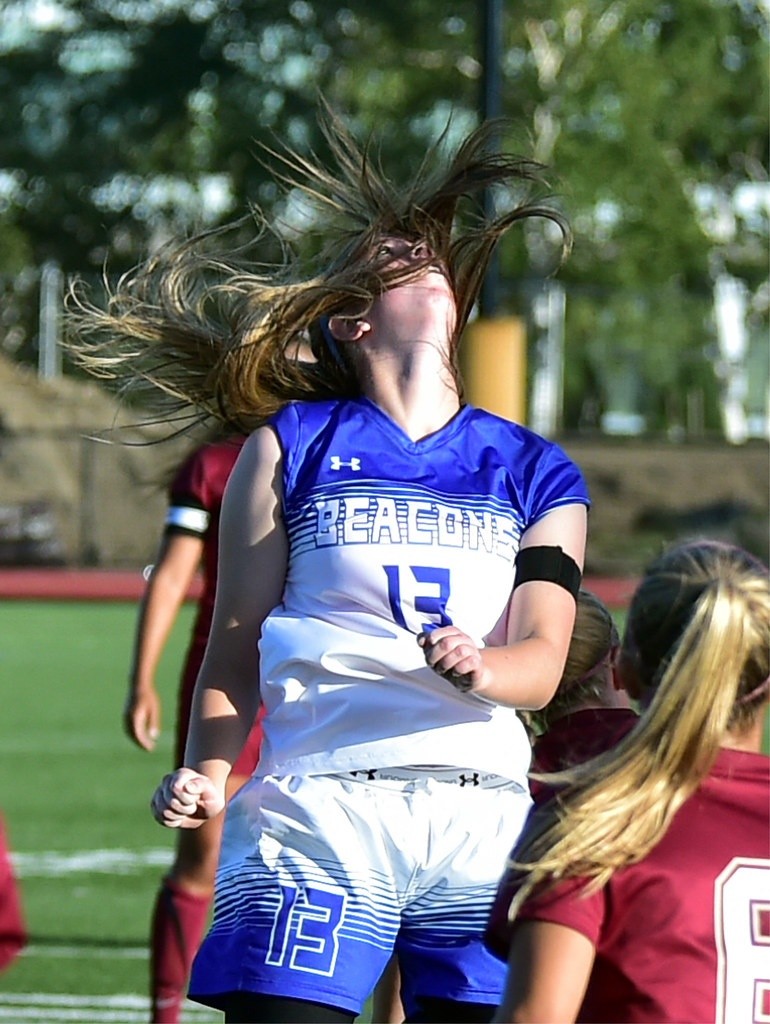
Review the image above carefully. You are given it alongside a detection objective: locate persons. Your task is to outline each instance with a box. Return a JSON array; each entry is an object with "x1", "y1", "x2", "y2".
[
  {"x1": 486, "y1": 537, "x2": 770, "y2": 1024},
  {"x1": 58, "y1": 89, "x2": 639, "y2": 1024}
]
